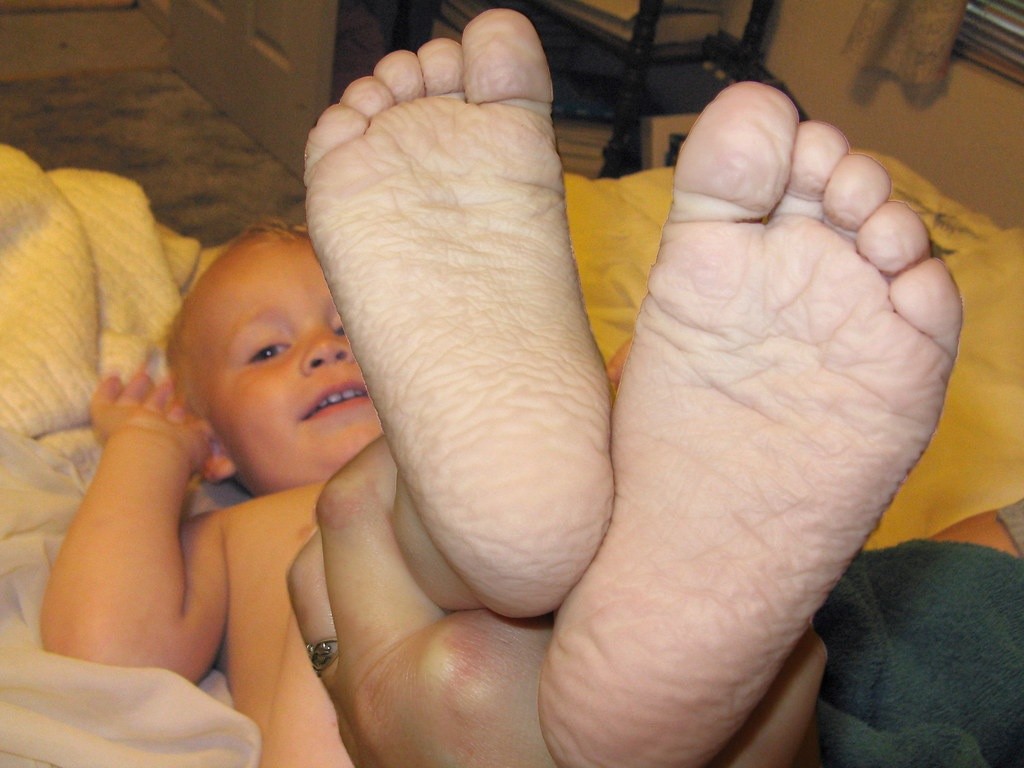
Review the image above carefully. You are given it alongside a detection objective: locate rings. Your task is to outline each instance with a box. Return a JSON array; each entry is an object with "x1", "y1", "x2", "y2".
[{"x1": 306, "y1": 638, "x2": 338, "y2": 678}]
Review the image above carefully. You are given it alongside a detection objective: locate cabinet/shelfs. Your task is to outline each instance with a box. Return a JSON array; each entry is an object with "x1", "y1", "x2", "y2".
[{"x1": 166, "y1": 0, "x2": 407, "y2": 188}]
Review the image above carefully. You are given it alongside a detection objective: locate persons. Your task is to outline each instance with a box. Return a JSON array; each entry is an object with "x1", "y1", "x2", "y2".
[
  {"x1": 288, "y1": 436, "x2": 826, "y2": 768},
  {"x1": 41, "y1": 10, "x2": 963, "y2": 768}
]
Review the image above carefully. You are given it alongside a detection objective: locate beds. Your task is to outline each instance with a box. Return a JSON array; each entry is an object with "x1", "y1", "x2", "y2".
[{"x1": 0, "y1": 141, "x2": 1024, "y2": 768}]
